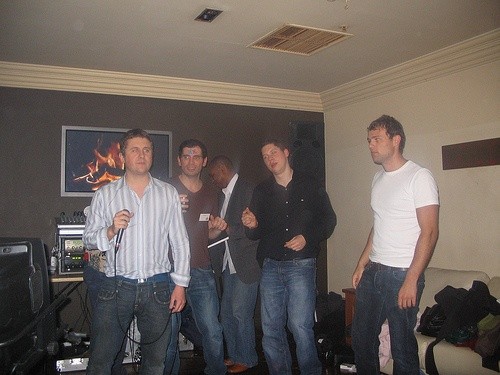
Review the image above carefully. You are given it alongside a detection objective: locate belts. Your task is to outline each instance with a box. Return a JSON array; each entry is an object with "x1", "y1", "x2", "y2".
[
  {"x1": 371, "y1": 262, "x2": 410, "y2": 272},
  {"x1": 117, "y1": 275, "x2": 168, "y2": 283}
]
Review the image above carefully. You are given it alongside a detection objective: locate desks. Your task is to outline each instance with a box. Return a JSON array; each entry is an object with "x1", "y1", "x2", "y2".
[{"x1": 47, "y1": 271, "x2": 85, "y2": 283}]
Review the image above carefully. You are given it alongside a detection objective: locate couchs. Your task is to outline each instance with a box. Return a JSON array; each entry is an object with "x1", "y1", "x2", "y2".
[{"x1": 378, "y1": 265, "x2": 500, "y2": 375}]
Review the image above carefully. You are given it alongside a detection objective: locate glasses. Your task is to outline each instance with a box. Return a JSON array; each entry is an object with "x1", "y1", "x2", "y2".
[
  {"x1": 125, "y1": 145, "x2": 154, "y2": 157},
  {"x1": 180, "y1": 154, "x2": 204, "y2": 161}
]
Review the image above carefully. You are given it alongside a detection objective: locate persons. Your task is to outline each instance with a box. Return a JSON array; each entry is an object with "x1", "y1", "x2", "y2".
[
  {"x1": 350, "y1": 114, "x2": 439, "y2": 375},
  {"x1": 242, "y1": 138, "x2": 337, "y2": 375},
  {"x1": 163, "y1": 139, "x2": 228, "y2": 375},
  {"x1": 82, "y1": 128, "x2": 191, "y2": 375},
  {"x1": 209, "y1": 155, "x2": 258, "y2": 373}
]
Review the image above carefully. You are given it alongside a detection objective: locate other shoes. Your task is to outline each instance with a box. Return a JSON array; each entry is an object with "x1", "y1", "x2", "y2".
[
  {"x1": 227, "y1": 364, "x2": 248, "y2": 373},
  {"x1": 223, "y1": 358, "x2": 234, "y2": 366}
]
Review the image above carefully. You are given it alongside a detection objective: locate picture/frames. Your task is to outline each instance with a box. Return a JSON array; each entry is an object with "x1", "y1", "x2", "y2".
[{"x1": 60, "y1": 125, "x2": 172, "y2": 198}]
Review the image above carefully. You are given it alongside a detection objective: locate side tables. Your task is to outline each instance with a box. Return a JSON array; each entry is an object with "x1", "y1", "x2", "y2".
[{"x1": 341, "y1": 286, "x2": 357, "y2": 347}]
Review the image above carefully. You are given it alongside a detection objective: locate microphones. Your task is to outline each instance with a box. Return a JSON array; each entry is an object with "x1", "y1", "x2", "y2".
[{"x1": 115, "y1": 209, "x2": 130, "y2": 251}]
